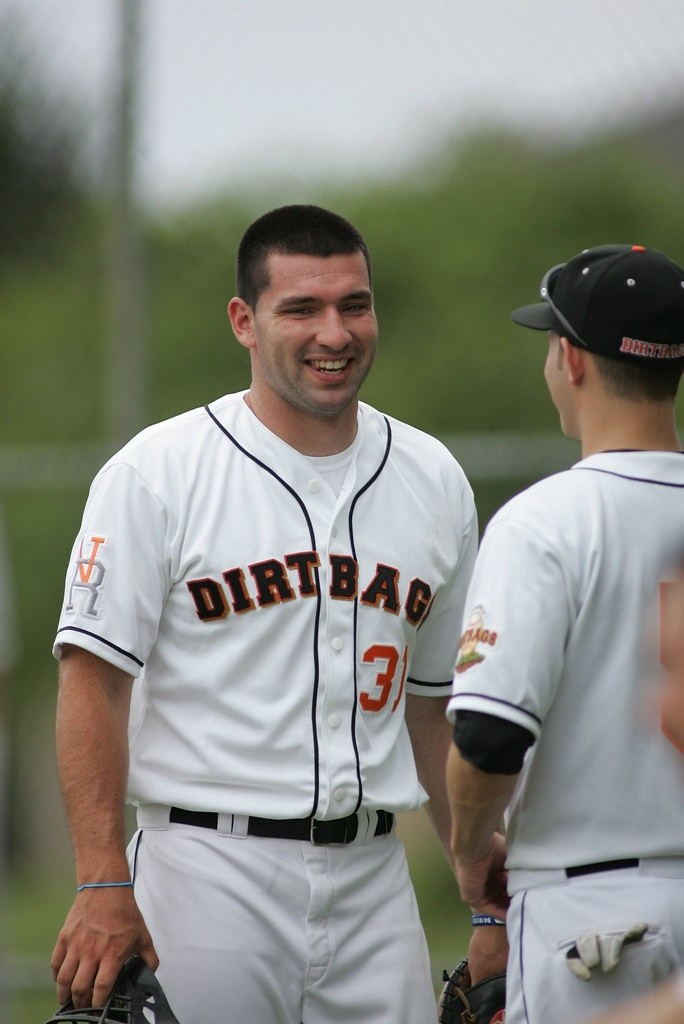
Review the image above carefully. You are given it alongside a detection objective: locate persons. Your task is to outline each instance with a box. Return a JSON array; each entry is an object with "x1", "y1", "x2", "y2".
[
  {"x1": 52, "y1": 204, "x2": 510, "y2": 1024},
  {"x1": 435, "y1": 241, "x2": 684, "y2": 1024}
]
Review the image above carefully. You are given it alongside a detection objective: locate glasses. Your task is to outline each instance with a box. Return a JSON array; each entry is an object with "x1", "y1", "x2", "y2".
[{"x1": 539, "y1": 261, "x2": 594, "y2": 350}]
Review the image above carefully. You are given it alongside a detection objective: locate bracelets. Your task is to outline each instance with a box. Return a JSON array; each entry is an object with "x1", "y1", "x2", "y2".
[
  {"x1": 469, "y1": 915, "x2": 505, "y2": 927},
  {"x1": 77, "y1": 881, "x2": 134, "y2": 892}
]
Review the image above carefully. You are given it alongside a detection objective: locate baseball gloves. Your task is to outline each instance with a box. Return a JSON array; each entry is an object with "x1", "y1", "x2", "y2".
[{"x1": 436, "y1": 958, "x2": 506, "y2": 1024}]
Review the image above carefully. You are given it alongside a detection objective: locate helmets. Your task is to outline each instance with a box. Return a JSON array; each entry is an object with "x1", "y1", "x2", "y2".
[{"x1": 44, "y1": 955, "x2": 179, "y2": 1024}]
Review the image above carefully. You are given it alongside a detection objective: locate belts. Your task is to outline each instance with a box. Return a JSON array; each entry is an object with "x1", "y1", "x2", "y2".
[
  {"x1": 565, "y1": 859, "x2": 639, "y2": 877},
  {"x1": 169, "y1": 807, "x2": 394, "y2": 847}
]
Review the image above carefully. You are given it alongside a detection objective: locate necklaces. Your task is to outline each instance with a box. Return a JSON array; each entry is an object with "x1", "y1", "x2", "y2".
[{"x1": 600, "y1": 448, "x2": 684, "y2": 454}]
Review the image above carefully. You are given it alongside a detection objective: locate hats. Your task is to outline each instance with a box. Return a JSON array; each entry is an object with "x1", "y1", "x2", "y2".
[{"x1": 512, "y1": 243, "x2": 684, "y2": 375}]
